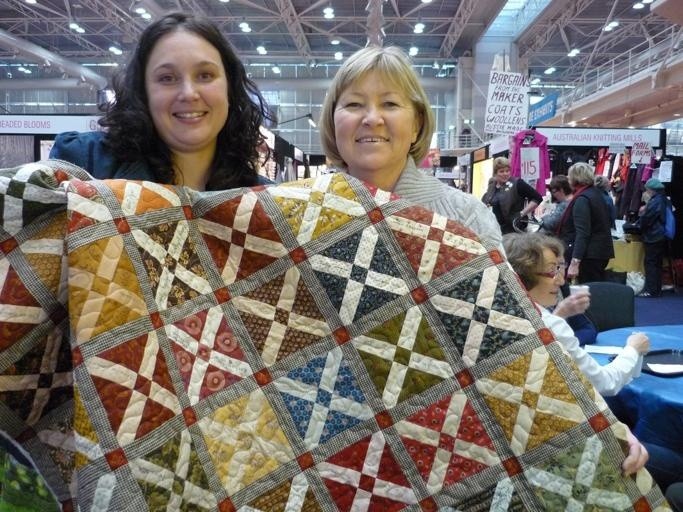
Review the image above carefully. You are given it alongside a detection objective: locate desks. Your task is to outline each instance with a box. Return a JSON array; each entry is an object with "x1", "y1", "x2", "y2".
[{"x1": 589, "y1": 324, "x2": 683, "y2": 456}]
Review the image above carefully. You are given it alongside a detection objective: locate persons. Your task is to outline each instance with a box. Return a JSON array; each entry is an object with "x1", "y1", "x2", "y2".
[
  {"x1": 318, "y1": 45, "x2": 650, "y2": 478},
  {"x1": 49, "y1": 10, "x2": 276, "y2": 193},
  {"x1": 482, "y1": 157, "x2": 672, "y2": 297},
  {"x1": 541, "y1": 236, "x2": 598, "y2": 346},
  {"x1": 502, "y1": 232, "x2": 648, "y2": 397}
]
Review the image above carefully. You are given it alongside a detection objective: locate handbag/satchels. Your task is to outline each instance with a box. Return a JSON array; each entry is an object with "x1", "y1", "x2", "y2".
[{"x1": 664, "y1": 206, "x2": 676, "y2": 240}]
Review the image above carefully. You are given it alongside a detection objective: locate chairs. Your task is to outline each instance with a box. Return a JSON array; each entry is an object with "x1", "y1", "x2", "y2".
[{"x1": 584, "y1": 281, "x2": 634, "y2": 332}]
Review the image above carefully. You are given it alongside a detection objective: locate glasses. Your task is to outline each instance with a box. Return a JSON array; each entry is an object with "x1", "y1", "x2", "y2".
[{"x1": 535, "y1": 267, "x2": 561, "y2": 278}]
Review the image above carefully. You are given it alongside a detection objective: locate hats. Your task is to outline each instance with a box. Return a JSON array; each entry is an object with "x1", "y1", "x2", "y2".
[{"x1": 647, "y1": 178, "x2": 664, "y2": 190}]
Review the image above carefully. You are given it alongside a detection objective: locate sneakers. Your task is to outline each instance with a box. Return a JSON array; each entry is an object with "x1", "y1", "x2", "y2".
[{"x1": 637, "y1": 289, "x2": 652, "y2": 298}]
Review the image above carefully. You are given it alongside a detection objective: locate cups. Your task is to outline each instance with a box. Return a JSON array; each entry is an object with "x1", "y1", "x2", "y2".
[
  {"x1": 570, "y1": 285, "x2": 590, "y2": 298},
  {"x1": 632, "y1": 355, "x2": 644, "y2": 378}
]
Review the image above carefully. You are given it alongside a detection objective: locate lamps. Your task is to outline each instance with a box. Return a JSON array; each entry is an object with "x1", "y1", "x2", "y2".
[{"x1": 277, "y1": 113, "x2": 317, "y2": 128}]
